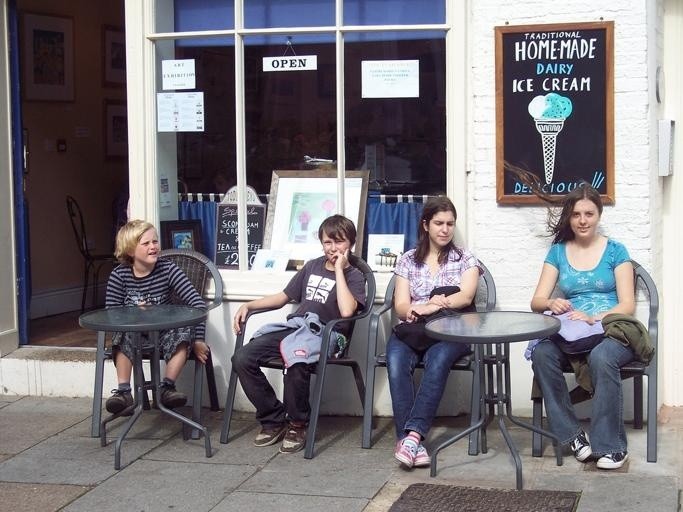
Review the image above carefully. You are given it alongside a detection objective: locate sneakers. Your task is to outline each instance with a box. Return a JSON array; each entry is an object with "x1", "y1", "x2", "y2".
[
  {"x1": 103, "y1": 387, "x2": 136, "y2": 418},
  {"x1": 278, "y1": 417, "x2": 309, "y2": 453},
  {"x1": 596, "y1": 448, "x2": 629, "y2": 468},
  {"x1": 253, "y1": 416, "x2": 288, "y2": 448},
  {"x1": 394, "y1": 433, "x2": 430, "y2": 469},
  {"x1": 155, "y1": 381, "x2": 188, "y2": 410},
  {"x1": 568, "y1": 428, "x2": 591, "y2": 462}
]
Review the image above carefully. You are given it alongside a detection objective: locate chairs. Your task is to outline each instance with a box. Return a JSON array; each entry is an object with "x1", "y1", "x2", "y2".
[
  {"x1": 89, "y1": 251, "x2": 225, "y2": 440},
  {"x1": 217, "y1": 255, "x2": 378, "y2": 462},
  {"x1": 525, "y1": 251, "x2": 660, "y2": 466},
  {"x1": 355, "y1": 248, "x2": 498, "y2": 458},
  {"x1": 64, "y1": 192, "x2": 121, "y2": 316}
]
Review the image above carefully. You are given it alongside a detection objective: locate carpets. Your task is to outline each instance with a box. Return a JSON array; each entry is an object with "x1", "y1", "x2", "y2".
[{"x1": 387, "y1": 483, "x2": 583, "y2": 512}]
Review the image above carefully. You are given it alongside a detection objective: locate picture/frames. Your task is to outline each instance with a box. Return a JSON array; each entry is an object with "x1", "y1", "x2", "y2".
[
  {"x1": 161, "y1": 217, "x2": 204, "y2": 258},
  {"x1": 101, "y1": 97, "x2": 128, "y2": 160},
  {"x1": 20, "y1": 10, "x2": 78, "y2": 105},
  {"x1": 101, "y1": 22, "x2": 127, "y2": 89},
  {"x1": 263, "y1": 168, "x2": 371, "y2": 261}
]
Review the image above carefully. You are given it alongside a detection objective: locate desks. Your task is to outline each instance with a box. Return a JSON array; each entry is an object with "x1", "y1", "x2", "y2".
[
  {"x1": 75, "y1": 304, "x2": 214, "y2": 470},
  {"x1": 422, "y1": 311, "x2": 567, "y2": 492}
]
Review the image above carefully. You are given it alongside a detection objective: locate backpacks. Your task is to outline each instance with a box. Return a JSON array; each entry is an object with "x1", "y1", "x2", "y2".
[{"x1": 390, "y1": 285, "x2": 476, "y2": 353}]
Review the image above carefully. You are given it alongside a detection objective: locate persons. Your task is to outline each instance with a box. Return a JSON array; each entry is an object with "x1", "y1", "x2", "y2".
[
  {"x1": 231, "y1": 213, "x2": 366, "y2": 458},
  {"x1": 530, "y1": 182, "x2": 635, "y2": 469},
  {"x1": 385, "y1": 192, "x2": 485, "y2": 471},
  {"x1": 101, "y1": 219, "x2": 209, "y2": 419}
]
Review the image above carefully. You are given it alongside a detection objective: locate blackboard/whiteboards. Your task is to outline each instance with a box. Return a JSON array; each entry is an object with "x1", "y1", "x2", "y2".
[{"x1": 214, "y1": 203, "x2": 266, "y2": 271}]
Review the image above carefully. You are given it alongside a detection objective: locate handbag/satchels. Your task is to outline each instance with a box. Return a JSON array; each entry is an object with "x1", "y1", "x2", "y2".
[{"x1": 544, "y1": 307, "x2": 605, "y2": 357}]
